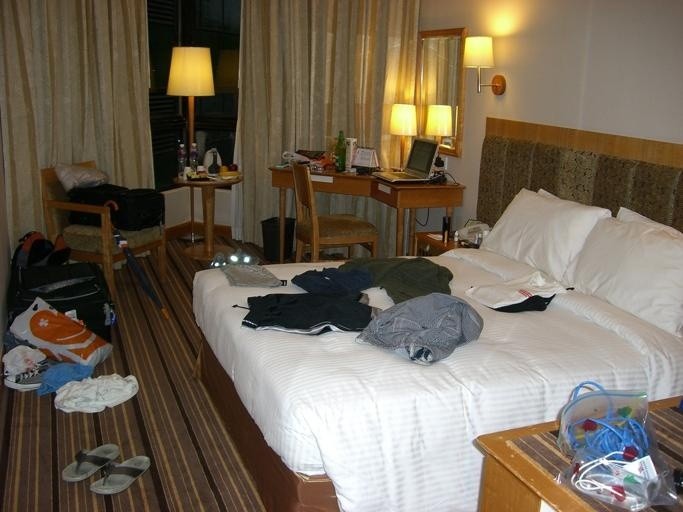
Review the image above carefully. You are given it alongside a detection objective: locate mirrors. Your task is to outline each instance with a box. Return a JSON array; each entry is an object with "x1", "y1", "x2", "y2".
[{"x1": 414, "y1": 27, "x2": 468, "y2": 158}]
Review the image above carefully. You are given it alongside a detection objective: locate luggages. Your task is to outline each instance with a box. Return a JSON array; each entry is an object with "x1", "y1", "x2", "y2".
[
  {"x1": 8, "y1": 261, "x2": 114, "y2": 336},
  {"x1": 66, "y1": 183, "x2": 165, "y2": 231}
]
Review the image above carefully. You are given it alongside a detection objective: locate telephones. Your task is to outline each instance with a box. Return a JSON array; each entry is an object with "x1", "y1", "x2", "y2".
[{"x1": 457, "y1": 223, "x2": 490, "y2": 242}]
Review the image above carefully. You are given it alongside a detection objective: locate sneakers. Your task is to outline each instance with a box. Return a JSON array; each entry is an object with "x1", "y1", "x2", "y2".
[
  {"x1": 36, "y1": 357, "x2": 65, "y2": 366},
  {"x1": 4, "y1": 363, "x2": 53, "y2": 391}
]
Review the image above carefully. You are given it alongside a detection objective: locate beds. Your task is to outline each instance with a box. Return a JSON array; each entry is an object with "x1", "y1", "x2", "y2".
[{"x1": 190, "y1": 115, "x2": 682, "y2": 512}]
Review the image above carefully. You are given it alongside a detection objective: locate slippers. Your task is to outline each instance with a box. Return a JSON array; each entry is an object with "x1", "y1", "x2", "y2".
[
  {"x1": 88, "y1": 454, "x2": 151, "y2": 496},
  {"x1": 60, "y1": 442, "x2": 121, "y2": 483}
]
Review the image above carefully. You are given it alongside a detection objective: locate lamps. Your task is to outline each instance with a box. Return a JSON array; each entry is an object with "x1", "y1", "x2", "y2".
[
  {"x1": 389, "y1": 103, "x2": 418, "y2": 172},
  {"x1": 425, "y1": 104, "x2": 452, "y2": 145},
  {"x1": 463, "y1": 36, "x2": 506, "y2": 96},
  {"x1": 166, "y1": 46, "x2": 215, "y2": 242}
]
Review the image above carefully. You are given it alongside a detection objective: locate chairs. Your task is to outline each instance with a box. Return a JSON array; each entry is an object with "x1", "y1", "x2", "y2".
[
  {"x1": 40, "y1": 160, "x2": 168, "y2": 294},
  {"x1": 292, "y1": 163, "x2": 379, "y2": 264}
]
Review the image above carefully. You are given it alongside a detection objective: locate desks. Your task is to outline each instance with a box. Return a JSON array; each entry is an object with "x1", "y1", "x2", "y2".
[
  {"x1": 267, "y1": 163, "x2": 467, "y2": 264},
  {"x1": 474, "y1": 394, "x2": 682, "y2": 512}
]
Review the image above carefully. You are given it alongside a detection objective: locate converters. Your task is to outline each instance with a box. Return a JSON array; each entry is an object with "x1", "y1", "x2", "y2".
[{"x1": 434, "y1": 160, "x2": 444, "y2": 167}]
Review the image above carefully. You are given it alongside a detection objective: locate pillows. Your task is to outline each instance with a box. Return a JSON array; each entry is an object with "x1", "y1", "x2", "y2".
[
  {"x1": 615, "y1": 206, "x2": 683, "y2": 239},
  {"x1": 561, "y1": 215, "x2": 683, "y2": 338},
  {"x1": 537, "y1": 187, "x2": 613, "y2": 217},
  {"x1": 478, "y1": 187, "x2": 608, "y2": 283}
]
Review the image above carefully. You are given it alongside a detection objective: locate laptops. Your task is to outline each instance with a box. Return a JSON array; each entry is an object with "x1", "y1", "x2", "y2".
[{"x1": 371, "y1": 135, "x2": 440, "y2": 183}]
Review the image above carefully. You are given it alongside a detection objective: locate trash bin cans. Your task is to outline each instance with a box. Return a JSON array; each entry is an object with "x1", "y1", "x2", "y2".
[{"x1": 260, "y1": 216, "x2": 296, "y2": 262}]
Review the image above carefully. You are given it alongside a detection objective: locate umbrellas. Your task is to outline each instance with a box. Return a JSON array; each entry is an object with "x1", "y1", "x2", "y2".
[{"x1": 103, "y1": 200, "x2": 171, "y2": 322}]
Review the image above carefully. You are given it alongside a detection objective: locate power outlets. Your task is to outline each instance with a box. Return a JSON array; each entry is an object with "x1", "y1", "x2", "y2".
[{"x1": 435, "y1": 157, "x2": 448, "y2": 171}]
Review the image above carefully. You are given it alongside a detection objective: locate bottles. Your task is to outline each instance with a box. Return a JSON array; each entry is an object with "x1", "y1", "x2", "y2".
[
  {"x1": 189, "y1": 143, "x2": 199, "y2": 174},
  {"x1": 334, "y1": 131, "x2": 347, "y2": 172},
  {"x1": 176, "y1": 144, "x2": 188, "y2": 176}
]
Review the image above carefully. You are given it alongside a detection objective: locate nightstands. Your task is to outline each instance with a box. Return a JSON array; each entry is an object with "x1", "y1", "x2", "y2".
[{"x1": 415, "y1": 229, "x2": 472, "y2": 257}]
